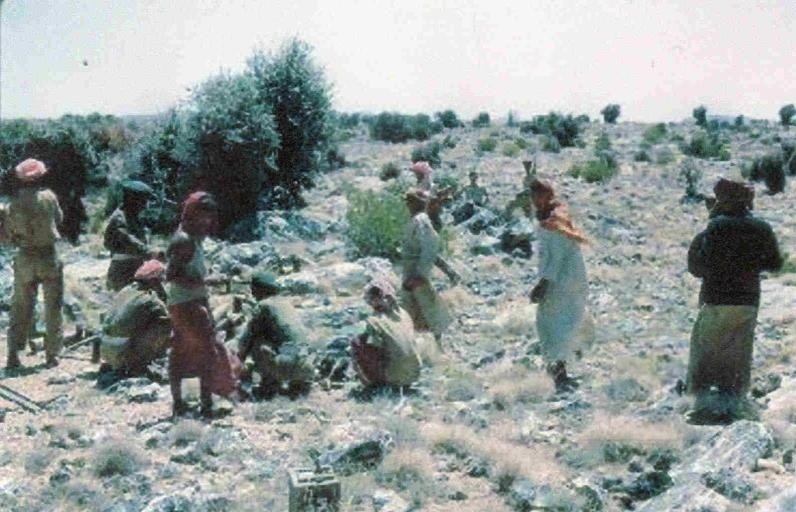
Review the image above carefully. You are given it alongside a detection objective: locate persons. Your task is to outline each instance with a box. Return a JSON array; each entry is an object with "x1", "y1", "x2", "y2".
[
  {"x1": 349, "y1": 280, "x2": 422, "y2": 398},
  {"x1": 229, "y1": 269, "x2": 313, "y2": 403},
  {"x1": 162, "y1": 186, "x2": 240, "y2": 421},
  {"x1": 396, "y1": 189, "x2": 452, "y2": 355},
  {"x1": 526, "y1": 177, "x2": 593, "y2": 397},
  {"x1": 2, "y1": 152, "x2": 64, "y2": 374},
  {"x1": 99, "y1": 180, "x2": 167, "y2": 303},
  {"x1": 406, "y1": 158, "x2": 466, "y2": 285},
  {"x1": 451, "y1": 170, "x2": 490, "y2": 208},
  {"x1": 683, "y1": 176, "x2": 786, "y2": 427},
  {"x1": 98, "y1": 258, "x2": 175, "y2": 378}
]
